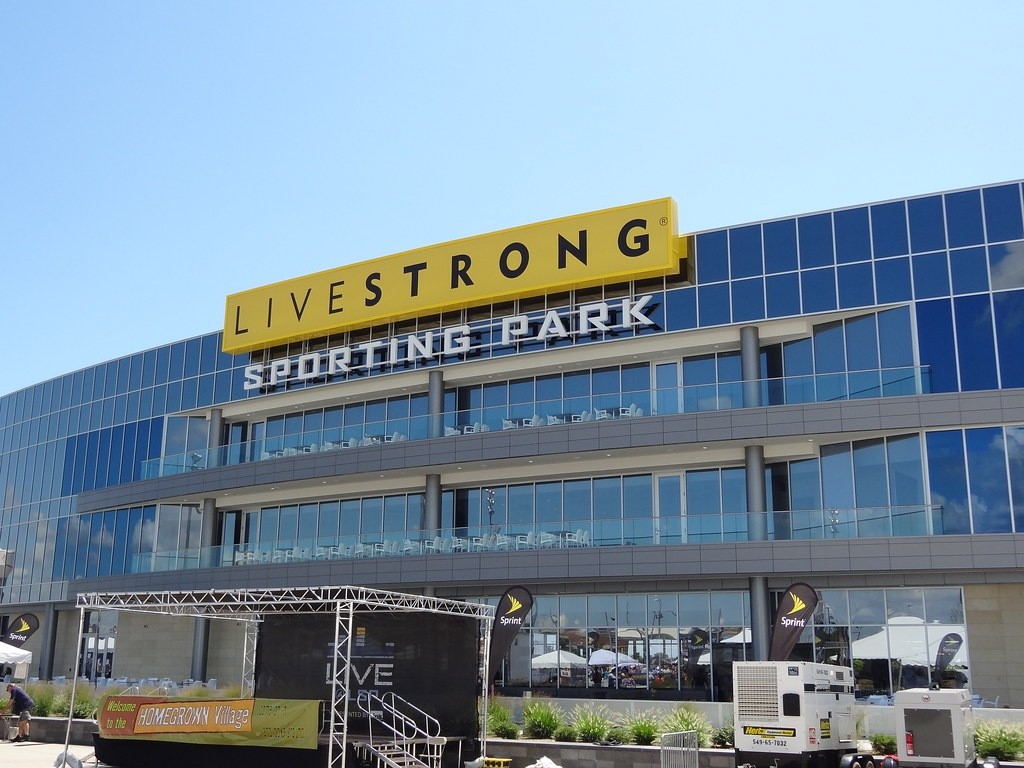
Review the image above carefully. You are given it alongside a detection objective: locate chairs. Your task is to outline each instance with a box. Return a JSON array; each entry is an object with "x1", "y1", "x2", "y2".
[
  {"x1": 234, "y1": 528, "x2": 591, "y2": 566},
  {"x1": 260, "y1": 431, "x2": 408, "y2": 461},
  {"x1": 444, "y1": 403, "x2": 643, "y2": 437},
  {"x1": 980, "y1": 695, "x2": 999, "y2": 708},
  {"x1": 27, "y1": 676, "x2": 217, "y2": 690}
]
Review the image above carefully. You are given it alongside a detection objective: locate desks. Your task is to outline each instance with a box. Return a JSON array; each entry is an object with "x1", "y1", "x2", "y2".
[
  {"x1": 97, "y1": 693, "x2": 344, "y2": 749},
  {"x1": 0, "y1": 714, "x2": 13, "y2": 743}
]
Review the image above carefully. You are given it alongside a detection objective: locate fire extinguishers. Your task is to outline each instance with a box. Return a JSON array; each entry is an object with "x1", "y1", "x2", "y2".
[{"x1": 906, "y1": 730, "x2": 914, "y2": 756}]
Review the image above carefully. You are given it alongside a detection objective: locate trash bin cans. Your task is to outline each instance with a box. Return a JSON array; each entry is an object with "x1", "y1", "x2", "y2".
[{"x1": 9, "y1": 716, "x2": 31, "y2": 739}]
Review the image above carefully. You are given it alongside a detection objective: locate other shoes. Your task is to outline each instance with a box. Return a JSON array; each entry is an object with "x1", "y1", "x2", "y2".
[
  {"x1": 22, "y1": 734, "x2": 29, "y2": 741},
  {"x1": 11, "y1": 735, "x2": 24, "y2": 742}
]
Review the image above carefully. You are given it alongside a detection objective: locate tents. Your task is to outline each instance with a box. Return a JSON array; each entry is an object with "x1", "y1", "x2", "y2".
[
  {"x1": 589, "y1": 649, "x2": 642, "y2": 666},
  {"x1": 0, "y1": 640, "x2": 32, "y2": 692},
  {"x1": 532, "y1": 649, "x2": 589, "y2": 669}
]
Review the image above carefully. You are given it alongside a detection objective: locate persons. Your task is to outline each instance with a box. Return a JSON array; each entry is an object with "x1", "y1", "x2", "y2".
[
  {"x1": 546, "y1": 667, "x2": 637, "y2": 691},
  {"x1": 3, "y1": 667, "x2": 12, "y2": 677},
  {"x1": 2, "y1": 684, "x2": 35, "y2": 742},
  {"x1": 655, "y1": 674, "x2": 662, "y2": 684}
]
